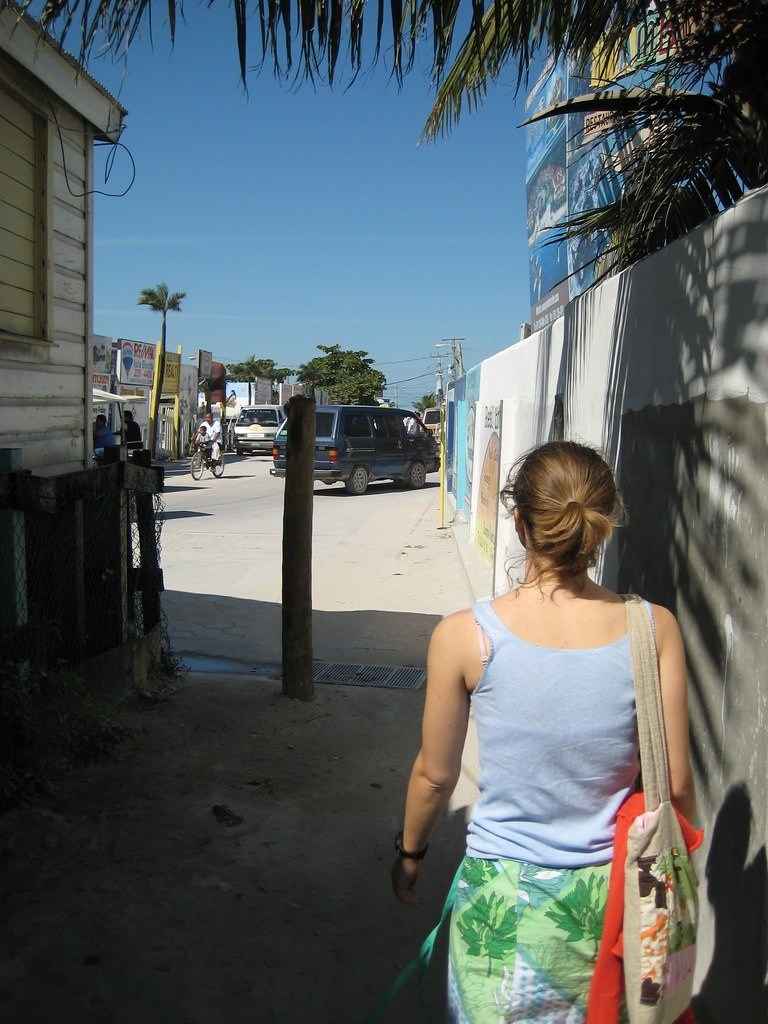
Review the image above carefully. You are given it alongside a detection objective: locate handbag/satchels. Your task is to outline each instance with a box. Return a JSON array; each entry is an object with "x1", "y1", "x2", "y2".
[{"x1": 619, "y1": 594, "x2": 699, "y2": 1024}]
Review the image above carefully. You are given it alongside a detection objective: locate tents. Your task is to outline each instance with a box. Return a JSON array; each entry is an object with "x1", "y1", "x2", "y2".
[{"x1": 92, "y1": 388, "x2": 128, "y2": 433}]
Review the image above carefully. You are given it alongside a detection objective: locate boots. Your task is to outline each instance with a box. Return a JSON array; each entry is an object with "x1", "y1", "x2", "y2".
[{"x1": 210, "y1": 460, "x2": 216, "y2": 472}]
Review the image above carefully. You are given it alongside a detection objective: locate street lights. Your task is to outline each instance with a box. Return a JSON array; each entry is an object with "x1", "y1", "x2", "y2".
[{"x1": 435, "y1": 343, "x2": 464, "y2": 374}]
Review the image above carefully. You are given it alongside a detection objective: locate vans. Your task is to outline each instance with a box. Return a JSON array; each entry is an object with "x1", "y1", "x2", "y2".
[
  {"x1": 269, "y1": 404, "x2": 441, "y2": 496},
  {"x1": 419, "y1": 407, "x2": 442, "y2": 457},
  {"x1": 233, "y1": 405, "x2": 286, "y2": 455}
]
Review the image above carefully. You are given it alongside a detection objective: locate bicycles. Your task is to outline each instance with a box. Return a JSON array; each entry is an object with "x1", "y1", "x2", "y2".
[{"x1": 189, "y1": 440, "x2": 225, "y2": 482}]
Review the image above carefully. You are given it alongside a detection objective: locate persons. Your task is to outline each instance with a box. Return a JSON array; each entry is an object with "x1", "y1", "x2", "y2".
[
  {"x1": 405, "y1": 411, "x2": 422, "y2": 434},
  {"x1": 392, "y1": 441, "x2": 697, "y2": 1024},
  {"x1": 94, "y1": 410, "x2": 223, "y2": 472}
]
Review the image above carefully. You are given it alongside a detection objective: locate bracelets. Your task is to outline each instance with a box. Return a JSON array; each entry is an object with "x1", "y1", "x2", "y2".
[{"x1": 394, "y1": 830, "x2": 429, "y2": 859}]
